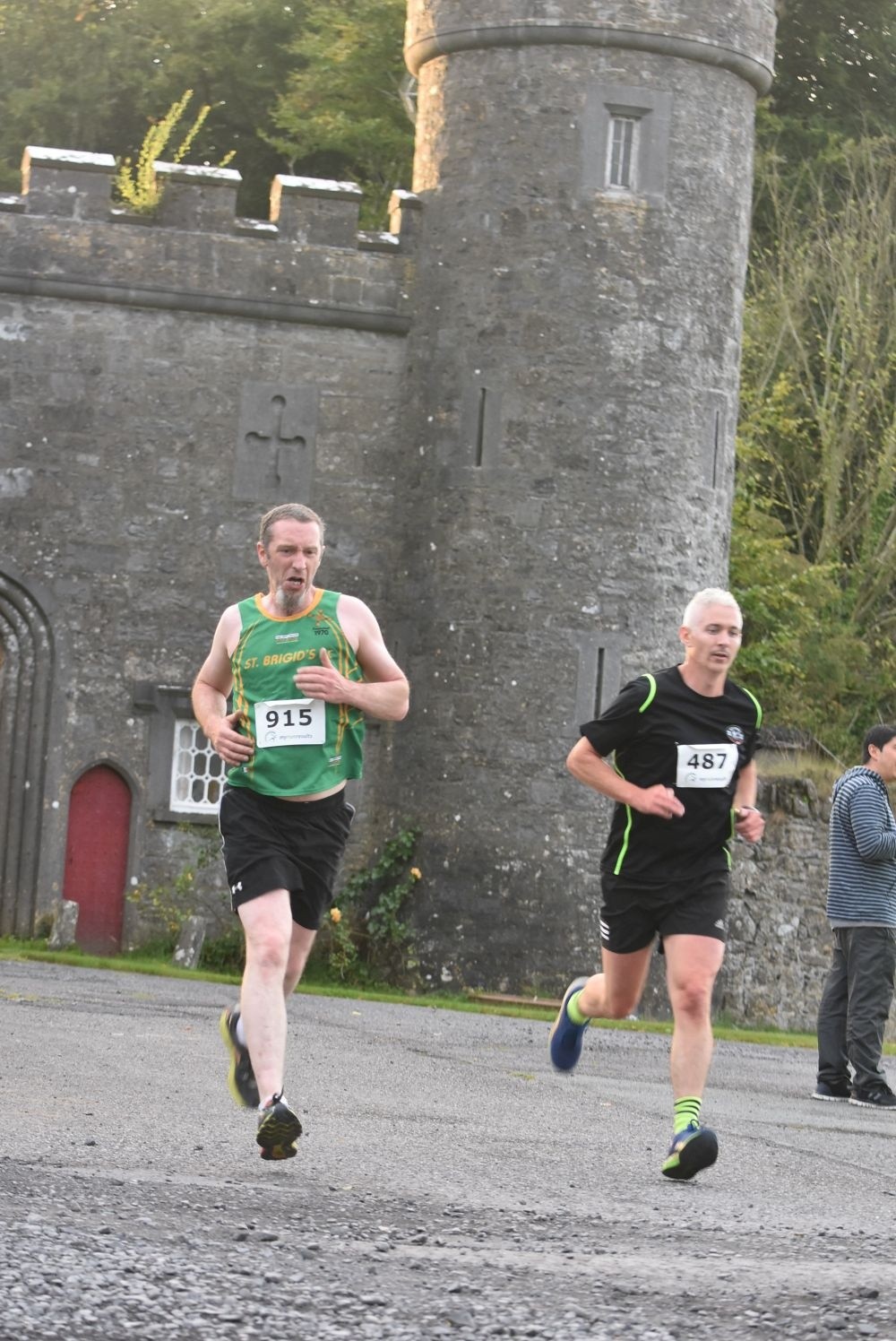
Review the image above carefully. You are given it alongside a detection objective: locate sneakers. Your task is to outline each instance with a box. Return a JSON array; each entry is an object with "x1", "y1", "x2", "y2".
[
  {"x1": 848, "y1": 1084, "x2": 896, "y2": 1110},
  {"x1": 548, "y1": 976, "x2": 591, "y2": 1071},
  {"x1": 662, "y1": 1119, "x2": 719, "y2": 1179},
  {"x1": 813, "y1": 1081, "x2": 852, "y2": 1101},
  {"x1": 219, "y1": 1008, "x2": 261, "y2": 1109},
  {"x1": 256, "y1": 1086, "x2": 303, "y2": 1161}
]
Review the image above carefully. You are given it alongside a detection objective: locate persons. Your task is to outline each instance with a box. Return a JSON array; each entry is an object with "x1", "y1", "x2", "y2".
[
  {"x1": 549, "y1": 587, "x2": 765, "y2": 1180},
  {"x1": 191, "y1": 505, "x2": 410, "y2": 1160},
  {"x1": 812, "y1": 725, "x2": 896, "y2": 1110}
]
